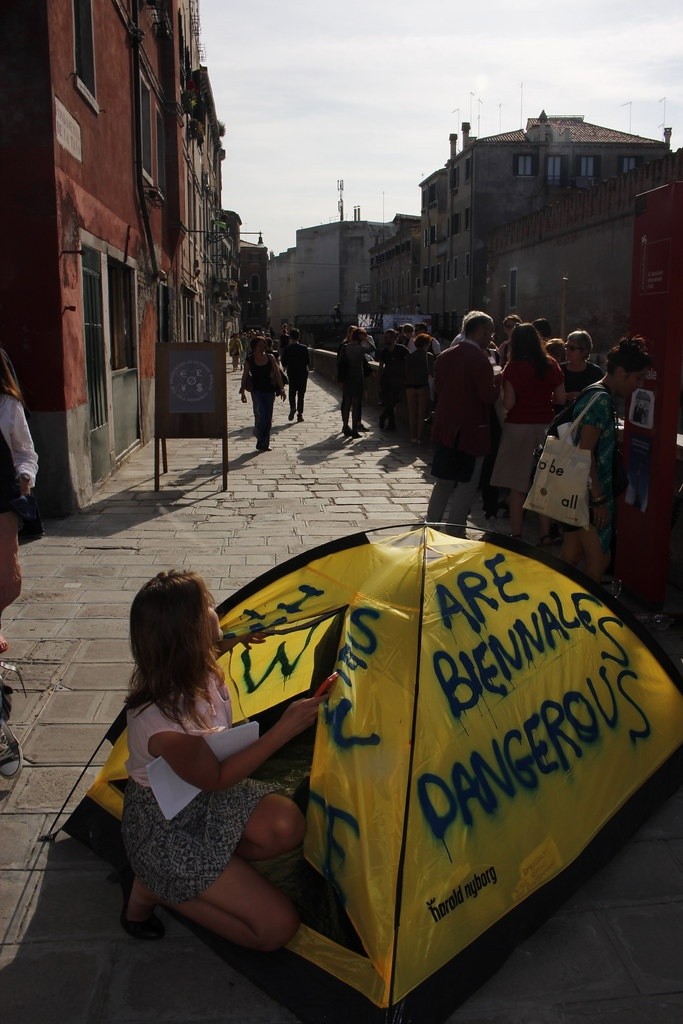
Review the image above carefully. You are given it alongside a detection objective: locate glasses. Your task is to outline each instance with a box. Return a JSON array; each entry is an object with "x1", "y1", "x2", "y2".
[{"x1": 565, "y1": 344, "x2": 582, "y2": 353}]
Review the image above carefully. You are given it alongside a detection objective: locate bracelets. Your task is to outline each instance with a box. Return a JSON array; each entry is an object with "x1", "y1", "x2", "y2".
[
  {"x1": 591, "y1": 495, "x2": 608, "y2": 506},
  {"x1": 20, "y1": 472, "x2": 30, "y2": 482}
]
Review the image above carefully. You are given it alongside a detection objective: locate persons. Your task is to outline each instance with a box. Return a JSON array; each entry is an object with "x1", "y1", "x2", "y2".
[
  {"x1": 119, "y1": 567, "x2": 330, "y2": 947},
  {"x1": 338, "y1": 311, "x2": 652, "y2": 583},
  {"x1": 282, "y1": 329, "x2": 310, "y2": 422},
  {"x1": 0, "y1": 349, "x2": 39, "y2": 496},
  {"x1": 0, "y1": 428, "x2": 27, "y2": 780},
  {"x1": 239, "y1": 337, "x2": 286, "y2": 451},
  {"x1": 229, "y1": 329, "x2": 290, "y2": 372}
]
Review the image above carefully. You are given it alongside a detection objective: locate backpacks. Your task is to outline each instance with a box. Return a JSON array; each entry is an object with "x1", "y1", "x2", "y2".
[{"x1": 529, "y1": 383, "x2": 611, "y2": 484}]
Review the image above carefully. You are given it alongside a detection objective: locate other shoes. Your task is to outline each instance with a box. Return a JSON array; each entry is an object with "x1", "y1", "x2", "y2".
[
  {"x1": 379, "y1": 415, "x2": 397, "y2": 432},
  {"x1": 256, "y1": 441, "x2": 271, "y2": 452},
  {"x1": 343, "y1": 423, "x2": 370, "y2": 439},
  {"x1": 289, "y1": 408, "x2": 303, "y2": 420}
]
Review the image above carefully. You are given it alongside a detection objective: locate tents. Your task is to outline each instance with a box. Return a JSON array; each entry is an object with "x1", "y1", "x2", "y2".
[{"x1": 38, "y1": 521, "x2": 680, "y2": 1024}]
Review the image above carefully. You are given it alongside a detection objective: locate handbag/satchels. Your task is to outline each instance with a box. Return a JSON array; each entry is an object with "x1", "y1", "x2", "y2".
[
  {"x1": 518, "y1": 434, "x2": 592, "y2": 533},
  {"x1": 275, "y1": 368, "x2": 288, "y2": 397},
  {"x1": 430, "y1": 442, "x2": 477, "y2": 482}
]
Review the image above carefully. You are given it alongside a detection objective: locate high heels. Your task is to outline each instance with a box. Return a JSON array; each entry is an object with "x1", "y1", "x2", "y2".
[{"x1": 119, "y1": 872, "x2": 148, "y2": 944}]
